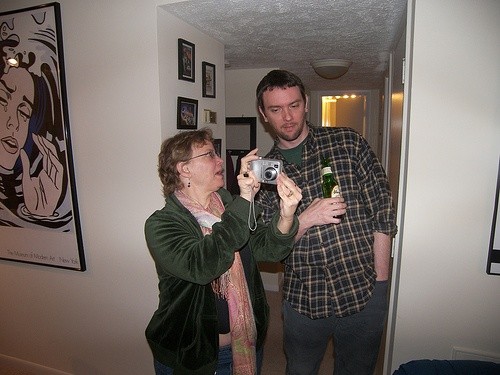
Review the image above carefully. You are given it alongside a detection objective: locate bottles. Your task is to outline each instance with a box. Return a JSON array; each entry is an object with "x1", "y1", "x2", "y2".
[{"x1": 319, "y1": 155, "x2": 344, "y2": 225}]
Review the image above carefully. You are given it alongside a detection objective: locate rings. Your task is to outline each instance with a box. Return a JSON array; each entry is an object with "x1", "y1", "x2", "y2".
[
  {"x1": 287, "y1": 191, "x2": 292, "y2": 197},
  {"x1": 243, "y1": 172, "x2": 249, "y2": 177}
]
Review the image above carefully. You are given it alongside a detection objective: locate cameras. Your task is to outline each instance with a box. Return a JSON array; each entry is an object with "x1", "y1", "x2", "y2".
[{"x1": 249, "y1": 159, "x2": 283, "y2": 184}]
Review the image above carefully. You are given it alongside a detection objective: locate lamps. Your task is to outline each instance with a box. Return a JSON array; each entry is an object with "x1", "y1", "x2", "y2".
[{"x1": 310, "y1": 58, "x2": 351, "y2": 79}]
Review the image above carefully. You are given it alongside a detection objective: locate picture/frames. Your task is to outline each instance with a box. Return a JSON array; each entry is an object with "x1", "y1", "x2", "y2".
[
  {"x1": 0, "y1": 1, "x2": 87, "y2": 272},
  {"x1": 177, "y1": 37, "x2": 195, "y2": 83},
  {"x1": 201, "y1": 61, "x2": 216, "y2": 98},
  {"x1": 177, "y1": 96, "x2": 198, "y2": 130}
]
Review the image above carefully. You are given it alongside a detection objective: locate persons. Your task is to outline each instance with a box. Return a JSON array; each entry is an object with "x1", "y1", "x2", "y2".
[
  {"x1": 145, "y1": 128, "x2": 302, "y2": 375},
  {"x1": 254, "y1": 70, "x2": 399, "y2": 375}
]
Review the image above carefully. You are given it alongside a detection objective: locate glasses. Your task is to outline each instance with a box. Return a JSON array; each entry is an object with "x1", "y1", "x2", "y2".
[{"x1": 179, "y1": 150, "x2": 218, "y2": 161}]
